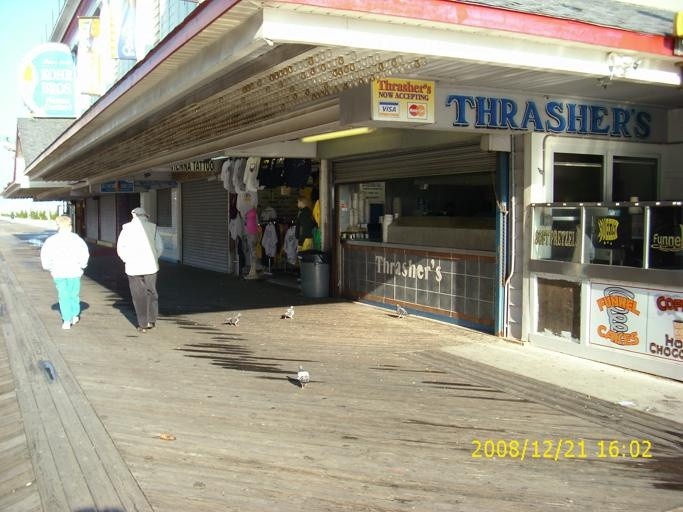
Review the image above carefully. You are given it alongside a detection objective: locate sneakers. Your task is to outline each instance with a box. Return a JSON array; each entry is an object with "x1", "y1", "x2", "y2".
[
  {"x1": 62, "y1": 320, "x2": 70, "y2": 329},
  {"x1": 71, "y1": 316, "x2": 79, "y2": 325}
]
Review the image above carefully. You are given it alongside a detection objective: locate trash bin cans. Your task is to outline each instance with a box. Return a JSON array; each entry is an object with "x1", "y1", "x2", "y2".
[{"x1": 297, "y1": 249, "x2": 329, "y2": 298}]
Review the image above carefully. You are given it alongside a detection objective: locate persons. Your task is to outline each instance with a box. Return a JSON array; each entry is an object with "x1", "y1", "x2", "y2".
[
  {"x1": 115, "y1": 207, "x2": 165, "y2": 332},
  {"x1": 39, "y1": 216, "x2": 90, "y2": 331},
  {"x1": 243, "y1": 208, "x2": 259, "y2": 277},
  {"x1": 293, "y1": 194, "x2": 315, "y2": 289}
]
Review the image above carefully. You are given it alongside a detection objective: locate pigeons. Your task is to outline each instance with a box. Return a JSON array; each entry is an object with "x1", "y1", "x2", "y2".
[
  {"x1": 282, "y1": 305, "x2": 295, "y2": 320},
  {"x1": 223, "y1": 312, "x2": 242, "y2": 326},
  {"x1": 298, "y1": 364, "x2": 310, "y2": 389},
  {"x1": 396, "y1": 303, "x2": 408, "y2": 319}
]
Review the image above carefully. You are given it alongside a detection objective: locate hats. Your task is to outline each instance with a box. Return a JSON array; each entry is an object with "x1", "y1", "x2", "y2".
[
  {"x1": 55, "y1": 216, "x2": 72, "y2": 225},
  {"x1": 131, "y1": 207, "x2": 149, "y2": 217}
]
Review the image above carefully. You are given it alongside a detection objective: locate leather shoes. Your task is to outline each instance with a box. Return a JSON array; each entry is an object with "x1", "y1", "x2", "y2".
[
  {"x1": 136, "y1": 325, "x2": 147, "y2": 333},
  {"x1": 146, "y1": 322, "x2": 153, "y2": 328}
]
